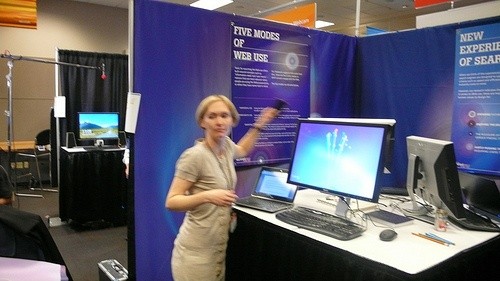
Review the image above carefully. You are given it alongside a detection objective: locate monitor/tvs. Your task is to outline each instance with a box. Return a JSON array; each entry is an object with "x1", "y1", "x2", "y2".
[
  {"x1": 404, "y1": 134, "x2": 466, "y2": 224},
  {"x1": 77, "y1": 111, "x2": 119, "y2": 146},
  {"x1": 286, "y1": 117, "x2": 398, "y2": 221}
]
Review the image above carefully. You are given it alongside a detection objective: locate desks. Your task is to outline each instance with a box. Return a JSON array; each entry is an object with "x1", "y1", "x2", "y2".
[
  {"x1": 0, "y1": 139, "x2": 48, "y2": 188},
  {"x1": 58, "y1": 146, "x2": 128, "y2": 232},
  {"x1": 225, "y1": 192, "x2": 500, "y2": 281}
]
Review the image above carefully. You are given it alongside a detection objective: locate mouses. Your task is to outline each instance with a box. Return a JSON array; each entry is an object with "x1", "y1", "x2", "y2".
[{"x1": 380, "y1": 230, "x2": 396, "y2": 241}]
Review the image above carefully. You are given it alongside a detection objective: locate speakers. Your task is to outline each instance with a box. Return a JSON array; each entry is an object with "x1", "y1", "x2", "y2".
[
  {"x1": 66, "y1": 132, "x2": 74, "y2": 148},
  {"x1": 117, "y1": 132, "x2": 127, "y2": 147}
]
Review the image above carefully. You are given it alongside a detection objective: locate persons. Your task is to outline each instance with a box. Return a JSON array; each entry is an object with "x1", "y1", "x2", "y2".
[
  {"x1": 0, "y1": 165, "x2": 13, "y2": 206},
  {"x1": 165, "y1": 95, "x2": 280, "y2": 281}
]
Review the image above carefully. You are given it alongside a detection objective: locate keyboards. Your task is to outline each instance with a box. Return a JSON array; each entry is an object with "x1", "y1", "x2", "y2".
[
  {"x1": 83, "y1": 145, "x2": 119, "y2": 151},
  {"x1": 277, "y1": 207, "x2": 367, "y2": 239},
  {"x1": 453, "y1": 208, "x2": 500, "y2": 231}
]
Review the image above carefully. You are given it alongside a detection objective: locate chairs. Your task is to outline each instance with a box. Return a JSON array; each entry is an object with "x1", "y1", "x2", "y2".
[{"x1": 14, "y1": 129, "x2": 59, "y2": 198}]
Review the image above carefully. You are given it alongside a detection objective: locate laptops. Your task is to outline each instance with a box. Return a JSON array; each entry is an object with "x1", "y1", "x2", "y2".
[{"x1": 234, "y1": 166, "x2": 301, "y2": 214}]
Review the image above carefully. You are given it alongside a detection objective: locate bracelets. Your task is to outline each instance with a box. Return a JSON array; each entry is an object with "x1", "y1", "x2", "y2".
[{"x1": 249, "y1": 123, "x2": 265, "y2": 132}]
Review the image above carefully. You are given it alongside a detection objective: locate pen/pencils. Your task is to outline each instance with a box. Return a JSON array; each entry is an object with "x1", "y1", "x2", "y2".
[{"x1": 411, "y1": 232, "x2": 455, "y2": 246}]
